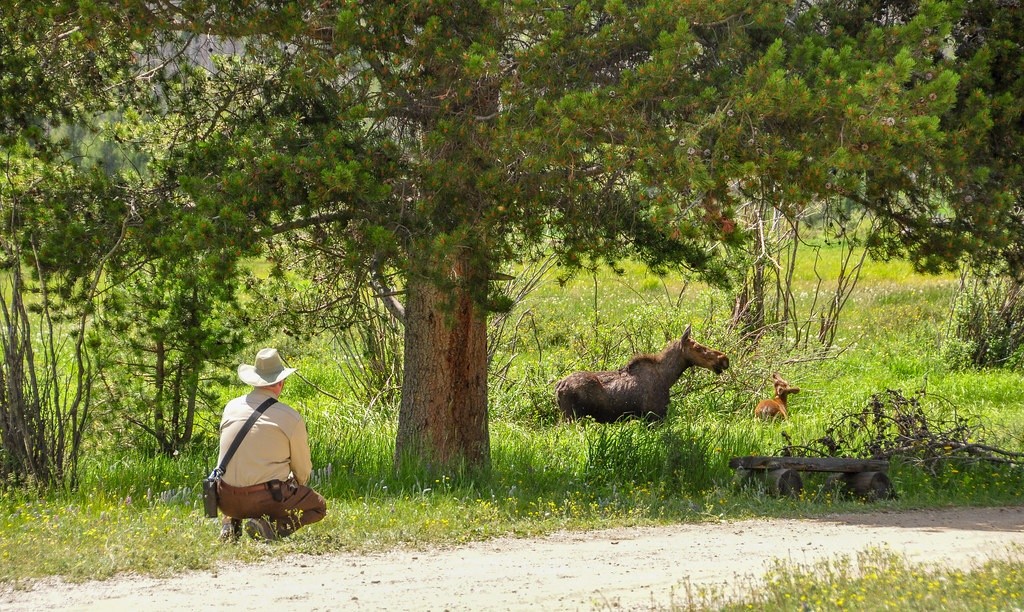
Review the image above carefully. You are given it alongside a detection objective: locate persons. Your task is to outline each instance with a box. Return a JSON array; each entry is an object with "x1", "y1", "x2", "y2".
[{"x1": 213, "y1": 347, "x2": 328, "y2": 543}]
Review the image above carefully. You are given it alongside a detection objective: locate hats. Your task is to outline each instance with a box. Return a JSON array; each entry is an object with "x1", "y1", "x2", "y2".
[{"x1": 236, "y1": 348, "x2": 298, "y2": 386}]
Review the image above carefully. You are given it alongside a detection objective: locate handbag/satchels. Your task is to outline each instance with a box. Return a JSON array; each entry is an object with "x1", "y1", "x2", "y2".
[{"x1": 202, "y1": 478, "x2": 218, "y2": 518}]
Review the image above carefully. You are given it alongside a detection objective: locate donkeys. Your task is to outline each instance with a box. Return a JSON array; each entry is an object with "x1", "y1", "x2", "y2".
[{"x1": 557, "y1": 323, "x2": 729, "y2": 427}]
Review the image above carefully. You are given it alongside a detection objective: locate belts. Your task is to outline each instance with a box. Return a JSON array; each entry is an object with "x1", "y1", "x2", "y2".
[{"x1": 221, "y1": 480, "x2": 269, "y2": 493}]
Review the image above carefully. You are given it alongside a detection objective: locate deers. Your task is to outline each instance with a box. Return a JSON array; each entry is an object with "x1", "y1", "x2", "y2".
[{"x1": 753, "y1": 373, "x2": 800, "y2": 421}]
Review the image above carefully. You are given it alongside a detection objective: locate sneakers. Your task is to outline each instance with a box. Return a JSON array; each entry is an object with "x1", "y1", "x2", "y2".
[
  {"x1": 220, "y1": 519, "x2": 242, "y2": 544},
  {"x1": 245, "y1": 518, "x2": 275, "y2": 542}
]
